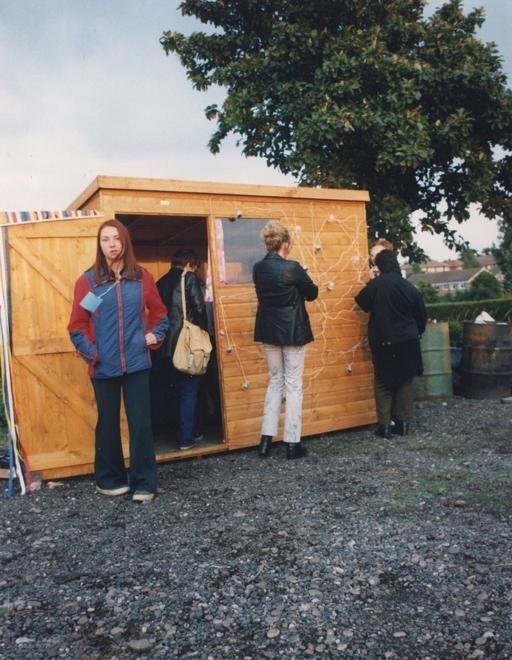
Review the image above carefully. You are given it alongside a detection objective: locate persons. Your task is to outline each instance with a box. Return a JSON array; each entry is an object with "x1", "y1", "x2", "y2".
[
  {"x1": 253, "y1": 221, "x2": 319, "y2": 459},
  {"x1": 355, "y1": 250, "x2": 427, "y2": 439},
  {"x1": 368, "y1": 239, "x2": 407, "y2": 280},
  {"x1": 68, "y1": 220, "x2": 170, "y2": 502},
  {"x1": 155, "y1": 248, "x2": 208, "y2": 450},
  {"x1": 196, "y1": 277, "x2": 220, "y2": 429}
]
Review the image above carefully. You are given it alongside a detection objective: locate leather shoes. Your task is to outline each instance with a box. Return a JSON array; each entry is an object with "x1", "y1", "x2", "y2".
[
  {"x1": 391, "y1": 421, "x2": 408, "y2": 436},
  {"x1": 374, "y1": 424, "x2": 391, "y2": 438},
  {"x1": 287, "y1": 443, "x2": 307, "y2": 458},
  {"x1": 258, "y1": 436, "x2": 271, "y2": 455}
]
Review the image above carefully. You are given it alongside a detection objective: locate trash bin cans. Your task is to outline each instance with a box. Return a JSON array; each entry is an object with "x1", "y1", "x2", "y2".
[{"x1": 412, "y1": 321, "x2": 454, "y2": 402}]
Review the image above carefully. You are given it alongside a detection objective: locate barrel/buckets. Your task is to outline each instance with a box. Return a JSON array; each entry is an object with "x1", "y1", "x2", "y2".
[{"x1": 420, "y1": 322, "x2": 452, "y2": 396}]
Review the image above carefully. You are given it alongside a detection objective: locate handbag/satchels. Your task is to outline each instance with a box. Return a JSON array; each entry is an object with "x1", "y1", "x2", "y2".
[{"x1": 173, "y1": 322, "x2": 212, "y2": 377}]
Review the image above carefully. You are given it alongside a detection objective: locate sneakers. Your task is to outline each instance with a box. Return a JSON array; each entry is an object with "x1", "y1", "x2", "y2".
[
  {"x1": 95, "y1": 484, "x2": 130, "y2": 496},
  {"x1": 133, "y1": 489, "x2": 154, "y2": 501},
  {"x1": 193, "y1": 433, "x2": 203, "y2": 441},
  {"x1": 180, "y1": 440, "x2": 196, "y2": 450}
]
